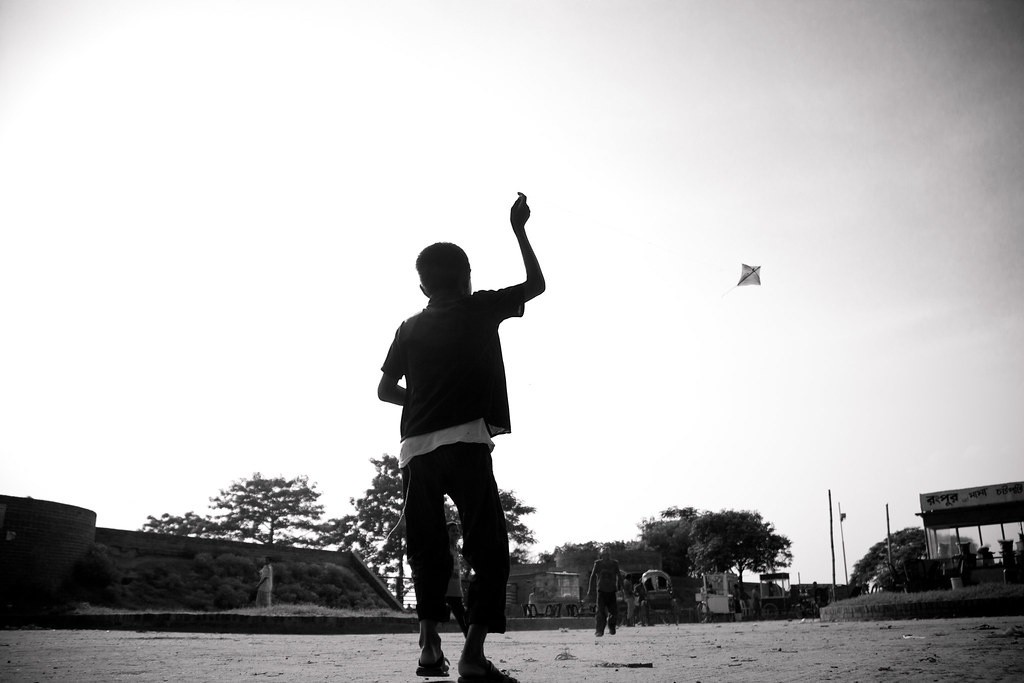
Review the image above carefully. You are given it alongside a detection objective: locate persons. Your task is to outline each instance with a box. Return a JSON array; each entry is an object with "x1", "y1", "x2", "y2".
[
  {"x1": 623, "y1": 573, "x2": 654, "y2": 626},
  {"x1": 417, "y1": 520, "x2": 470, "y2": 647},
  {"x1": 377, "y1": 191, "x2": 547, "y2": 683},
  {"x1": 707, "y1": 583, "x2": 716, "y2": 594},
  {"x1": 587, "y1": 546, "x2": 622, "y2": 636},
  {"x1": 255, "y1": 557, "x2": 273, "y2": 607},
  {"x1": 527, "y1": 587, "x2": 535, "y2": 616},
  {"x1": 732, "y1": 580, "x2": 818, "y2": 621}
]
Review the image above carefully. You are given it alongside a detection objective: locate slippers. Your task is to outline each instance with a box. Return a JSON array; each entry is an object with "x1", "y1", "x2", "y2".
[
  {"x1": 457, "y1": 660, "x2": 518, "y2": 683},
  {"x1": 416, "y1": 652, "x2": 450, "y2": 677}
]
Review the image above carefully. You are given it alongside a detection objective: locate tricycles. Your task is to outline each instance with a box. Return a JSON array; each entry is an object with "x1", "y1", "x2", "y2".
[
  {"x1": 759, "y1": 573, "x2": 818, "y2": 620},
  {"x1": 695, "y1": 573, "x2": 755, "y2": 623}
]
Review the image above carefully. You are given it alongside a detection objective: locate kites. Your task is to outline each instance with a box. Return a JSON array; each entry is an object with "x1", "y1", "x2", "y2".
[{"x1": 736, "y1": 263, "x2": 761, "y2": 286}]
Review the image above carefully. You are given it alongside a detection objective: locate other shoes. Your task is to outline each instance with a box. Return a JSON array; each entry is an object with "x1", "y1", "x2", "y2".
[
  {"x1": 607, "y1": 625, "x2": 616, "y2": 634},
  {"x1": 596, "y1": 632, "x2": 603, "y2": 637}
]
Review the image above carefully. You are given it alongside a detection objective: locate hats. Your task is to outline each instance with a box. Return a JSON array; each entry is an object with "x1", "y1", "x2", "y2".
[{"x1": 600, "y1": 546, "x2": 610, "y2": 556}]
